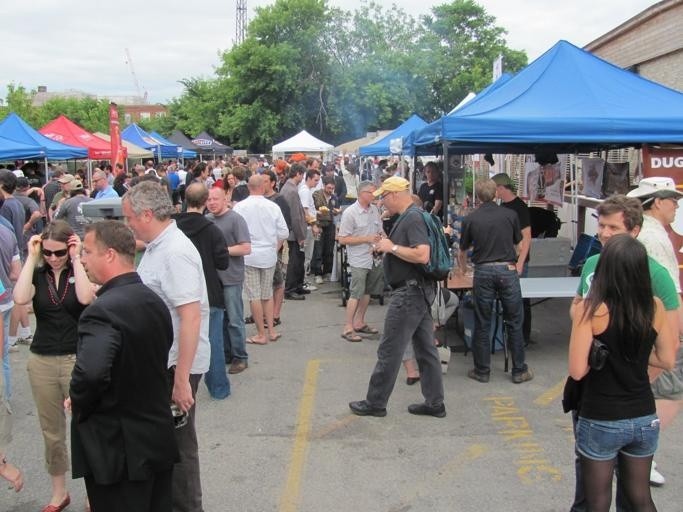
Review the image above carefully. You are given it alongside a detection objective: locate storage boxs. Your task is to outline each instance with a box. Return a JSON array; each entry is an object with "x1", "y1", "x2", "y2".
[{"x1": 525, "y1": 237, "x2": 572, "y2": 266}]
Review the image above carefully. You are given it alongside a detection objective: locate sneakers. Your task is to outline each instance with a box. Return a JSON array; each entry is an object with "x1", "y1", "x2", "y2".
[
  {"x1": 649, "y1": 460, "x2": 665, "y2": 485},
  {"x1": 407, "y1": 403, "x2": 446, "y2": 418},
  {"x1": 7, "y1": 343, "x2": 19, "y2": 353},
  {"x1": 511, "y1": 367, "x2": 533, "y2": 384},
  {"x1": 314, "y1": 274, "x2": 324, "y2": 285},
  {"x1": 466, "y1": 368, "x2": 489, "y2": 382},
  {"x1": 303, "y1": 281, "x2": 320, "y2": 291},
  {"x1": 349, "y1": 401, "x2": 388, "y2": 417},
  {"x1": 16, "y1": 334, "x2": 33, "y2": 345}
]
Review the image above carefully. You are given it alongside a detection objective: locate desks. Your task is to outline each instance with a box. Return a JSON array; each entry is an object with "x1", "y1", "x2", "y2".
[
  {"x1": 436, "y1": 270, "x2": 474, "y2": 356},
  {"x1": 490, "y1": 274, "x2": 612, "y2": 373}
]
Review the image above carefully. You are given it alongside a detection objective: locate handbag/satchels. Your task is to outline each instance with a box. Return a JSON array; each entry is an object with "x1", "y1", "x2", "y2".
[{"x1": 588, "y1": 338, "x2": 608, "y2": 371}]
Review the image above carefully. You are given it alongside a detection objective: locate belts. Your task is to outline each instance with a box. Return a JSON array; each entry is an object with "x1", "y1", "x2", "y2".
[
  {"x1": 389, "y1": 279, "x2": 422, "y2": 290},
  {"x1": 478, "y1": 261, "x2": 515, "y2": 266}
]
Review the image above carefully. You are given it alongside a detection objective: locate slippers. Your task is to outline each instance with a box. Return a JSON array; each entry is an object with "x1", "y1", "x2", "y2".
[
  {"x1": 245, "y1": 335, "x2": 267, "y2": 344},
  {"x1": 269, "y1": 333, "x2": 281, "y2": 341},
  {"x1": 354, "y1": 324, "x2": 378, "y2": 334},
  {"x1": 340, "y1": 330, "x2": 362, "y2": 342}
]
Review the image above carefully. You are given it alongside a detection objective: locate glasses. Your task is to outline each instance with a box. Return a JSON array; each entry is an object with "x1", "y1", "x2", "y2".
[
  {"x1": 379, "y1": 192, "x2": 392, "y2": 201},
  {"x1": 40, "y1": 248, "x2": 68, "y2": 257}
]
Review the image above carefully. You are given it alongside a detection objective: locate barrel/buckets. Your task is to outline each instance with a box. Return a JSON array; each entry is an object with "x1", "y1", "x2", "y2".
[
  {"x1": 459, "y1": 299, "x2": 508, "y2": 351},
  {"x1": 568, "y1": 232, "x2": 602, "y2": 274}
]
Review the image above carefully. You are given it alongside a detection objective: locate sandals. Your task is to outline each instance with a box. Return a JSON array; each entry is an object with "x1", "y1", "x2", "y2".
[
  {"x1": 243, "y1": 314, "x2": 256, "y2": 325},
  {"x1": 262, "y1": 317, "x2": 281, "y2": 328}
]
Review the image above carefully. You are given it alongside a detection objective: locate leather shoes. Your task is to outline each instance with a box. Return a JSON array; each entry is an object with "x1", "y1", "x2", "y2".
[
  {"x1": 406, "y1": 375, "x2": 420, "y2": 386},
  {"x1": 283, "y1": 291, "x2": 305, "y2": 302},
  {"x1": 40, "y1": 490, "x2": 70, "y2": 512},
  {"x1": 295, "y1": 288, "x2": 311, "y2": 296},
  {"x1": 228, "y1": 359, "x2": 248, "y2": 374}
]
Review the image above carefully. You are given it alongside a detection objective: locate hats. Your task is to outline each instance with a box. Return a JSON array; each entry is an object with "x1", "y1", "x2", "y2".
[
  {"x1": 624, "y1": 175, "x2": 683, "y2": 201},
  {"x1": 69, "y1": 180, "x2": 83, "y2": 191},
  {"x1": 56, "y1": 174, "x2": 74, "y2": 185},
  {"x1": 371, "y1": 176, "x2": 410, "y2": 198},
  {"x1": 492, "y1": 173, "x2": 511, "y2": 186}
]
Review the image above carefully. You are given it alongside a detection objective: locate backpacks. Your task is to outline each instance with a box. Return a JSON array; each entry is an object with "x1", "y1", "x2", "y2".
[{"x1": 386, "y1": 208, "x2": 451, "y2": 282}]
